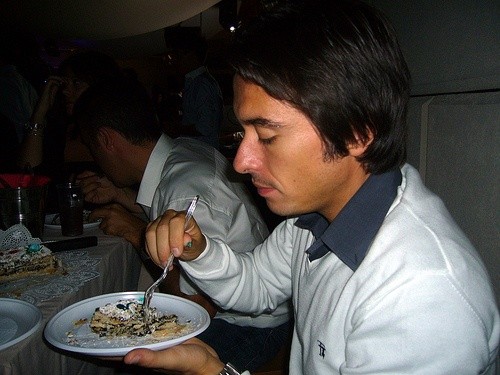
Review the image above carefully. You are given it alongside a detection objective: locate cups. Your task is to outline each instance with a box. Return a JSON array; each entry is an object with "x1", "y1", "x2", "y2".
[
  {"x1": 0, "y1": 185, "x2": 47, "y2": 242},
  {"x1": 57, "y1": 183, "x2": 84, "y2": 236}
]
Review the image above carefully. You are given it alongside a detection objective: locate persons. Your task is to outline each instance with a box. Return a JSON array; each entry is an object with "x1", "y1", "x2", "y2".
[
  {"x1": 17, "y1": 32, "x2": 295, "y2": 372},
  {"x1": 97, "y1": 0, "x2": 500, "y2": 375}
]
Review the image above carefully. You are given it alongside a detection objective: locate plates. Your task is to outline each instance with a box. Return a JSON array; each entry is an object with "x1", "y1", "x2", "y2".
[
  {"x1": 44, "y1": 210, "x2": 104, "y2": 231},
  {"x1": 44, "y1": 290, "x2": 211, "y2": 357},
  {"x1": 0, "y1": 297, "x2": 42, "y2": 351}
]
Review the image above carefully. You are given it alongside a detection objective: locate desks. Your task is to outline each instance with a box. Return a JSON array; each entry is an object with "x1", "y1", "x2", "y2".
[{"x1": 0, "y1": 216, "x2": 141, "y2": 375}]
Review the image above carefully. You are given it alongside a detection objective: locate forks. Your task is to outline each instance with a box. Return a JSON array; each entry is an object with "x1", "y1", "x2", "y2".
[{"x1": 143, "y1": 194, "x2": 201, "y2": 307}]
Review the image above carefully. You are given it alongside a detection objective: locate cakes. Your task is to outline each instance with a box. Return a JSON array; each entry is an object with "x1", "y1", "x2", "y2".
[
  {"x1": 89, "y1": 299, "x2": 178, "y2": 337},
  {"x1": 0, "y1": 242, "x2": 65, "y2": 283}
]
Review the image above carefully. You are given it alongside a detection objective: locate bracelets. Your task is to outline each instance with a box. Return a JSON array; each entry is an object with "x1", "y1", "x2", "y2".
[
  {"x1": 29, "y1": 120, "x2": 48, "y2": 136},
  {"x1": 219, "y1": 361, "x2": 240, "y2": 375}
]
